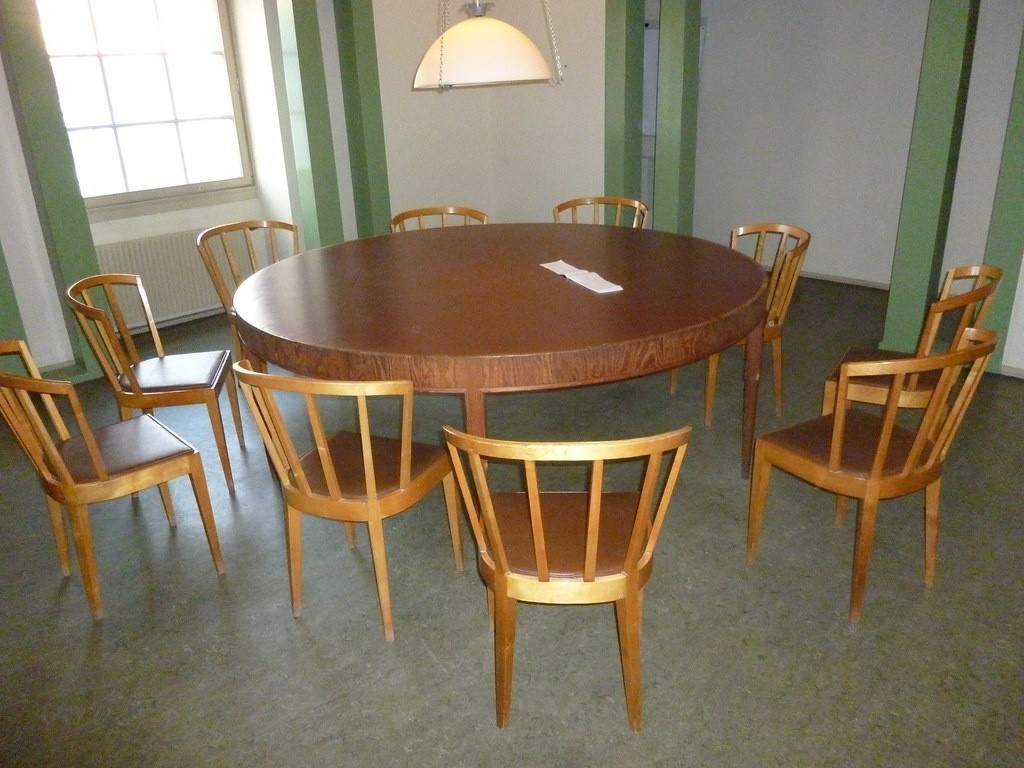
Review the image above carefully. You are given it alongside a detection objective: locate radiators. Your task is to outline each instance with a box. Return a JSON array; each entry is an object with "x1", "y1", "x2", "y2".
[{"x1": 95, "y1": 229, "x2": 254, "y2": 333}]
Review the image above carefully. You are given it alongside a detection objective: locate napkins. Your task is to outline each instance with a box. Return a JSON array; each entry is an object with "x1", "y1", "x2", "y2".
[{"x1": 539, "y1": 259, "x2": 624, "y2": 294}]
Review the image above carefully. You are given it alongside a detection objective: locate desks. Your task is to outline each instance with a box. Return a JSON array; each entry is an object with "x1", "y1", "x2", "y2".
[{"x1": 231, "y1": 224, "x2": 770, "y2": 482}]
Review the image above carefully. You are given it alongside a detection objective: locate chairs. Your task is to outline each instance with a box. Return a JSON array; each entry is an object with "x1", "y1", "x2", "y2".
[
  {"x1": 745, "y1": 327, "x2": 1000, "y2": 624},
  {"x1": 0, "y1": 340, "x2": 227, "y2": 623},
  {"x1": 442, "y1": 424, "x2": 692, "y2": 733},
  {"x1": 232, "y1": 359, "x2": 464, "y2": 643},
  {"x1": 553, "y1": 196, "x2": 650, "y2": 229},
  {"x1": 669, "y1": 222, "x2": 811, "y2": 431},
  {"x1": 390, "y1": 206, "x2": 488, "y2": 233},
  {"x1": 822, "y1": 265, "x2": 1003, "y2": 417},
  {"x1": 65, "y1": 272, "x2": 245, "y2": 500},
  {"x1": 196, "y1": 219, "x2": 299, "y2": 388}
]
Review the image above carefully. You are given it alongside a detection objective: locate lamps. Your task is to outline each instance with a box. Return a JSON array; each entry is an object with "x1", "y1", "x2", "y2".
[{"x1": 414, "y1": 0, "x2": 566, "y2": 92}]
